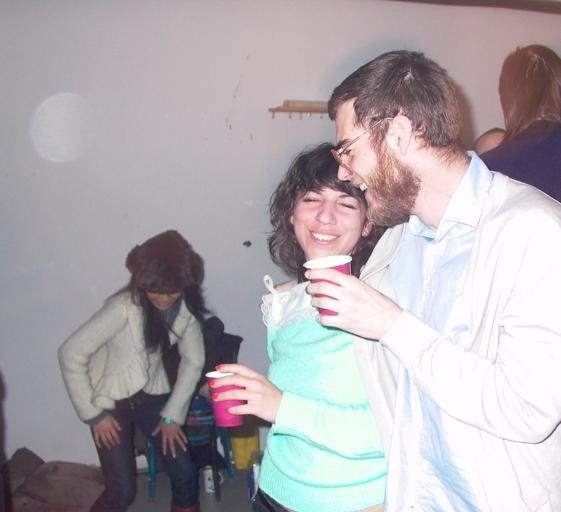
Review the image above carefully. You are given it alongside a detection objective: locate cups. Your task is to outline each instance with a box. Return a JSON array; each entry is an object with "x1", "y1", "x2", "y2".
[
  {"x1": 302, "y1": 254, "x2": 353, "y2": 316},
  {"x1": 206, "y1": 371, "x2": 247, "y2": 428},
  {"x1": 201, "y1": 465, "x2": 224, "y2": 496}
]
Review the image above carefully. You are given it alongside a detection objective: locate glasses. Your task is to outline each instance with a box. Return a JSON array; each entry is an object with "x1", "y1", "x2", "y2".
[{"x1": 330, "y1": 117, "x2": 394, "y2": 166}]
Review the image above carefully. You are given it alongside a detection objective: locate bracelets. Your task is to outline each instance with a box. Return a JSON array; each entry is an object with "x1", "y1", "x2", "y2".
[{"x1": 163, "y1": 417, "x2": 174, "y2": 425}]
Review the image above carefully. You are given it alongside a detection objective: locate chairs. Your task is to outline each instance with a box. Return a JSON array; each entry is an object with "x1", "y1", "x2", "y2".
[{"x1": 149, "y1": 332, "x2": 244, "y2": 501}]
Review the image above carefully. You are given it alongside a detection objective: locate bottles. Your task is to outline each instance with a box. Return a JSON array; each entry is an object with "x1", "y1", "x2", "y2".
[{"x1": 248, "y1": 449, "x2": 262, "y2": 503}]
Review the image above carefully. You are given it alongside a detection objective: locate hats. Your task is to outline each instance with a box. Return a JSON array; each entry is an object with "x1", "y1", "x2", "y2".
[{"x1": 125, "y1": 230, "x2": 204, "y2": 294}]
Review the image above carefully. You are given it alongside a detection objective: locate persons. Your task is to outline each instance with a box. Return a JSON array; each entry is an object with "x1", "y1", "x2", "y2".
[
  {"x1": 304, "y1": 50, "x2": 561, "y2": 512},
  {"x1": 208, "y1": 141, "x2": 378, "y2": 512},
  {"x1": 473, "y1": 44, "x2": 561, "y2": 203},
  {"x1": 57, "y1": 230, "x2": 205, "y2": 512}
]
